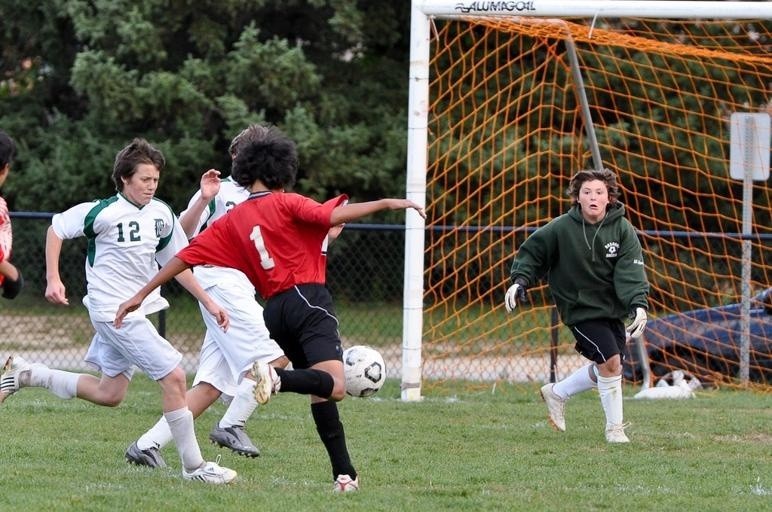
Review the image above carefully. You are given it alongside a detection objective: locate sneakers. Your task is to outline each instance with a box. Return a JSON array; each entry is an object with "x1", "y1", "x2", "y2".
[
  {"x1": 0, "y1": 356, "x2": 28, "y2": 402},
  {"x1": 209, "y1": 422, "x2": 260, "y2": 459},
  {"x1": 540, "y1": 382, "x2": 565, "y2": 431},
  {"x1": 333, "y1": 474, "x2": 359, "y2": 491},
  {"x1": 605, "y1": 421, "x2": 631, "y2": 443},
  {"x1": 125, "y1": 439, "x2": 166, "y2": 470},
  {"x1": 181, "y1": 454, "x2": 236, "y2": 485},
  {"x1": 251, "y1": 360, "x2": 280, "y2": 405}
]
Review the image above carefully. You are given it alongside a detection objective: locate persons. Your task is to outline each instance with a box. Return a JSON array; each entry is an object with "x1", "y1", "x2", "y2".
[
  {"x1": 113, "y1": 126, "x2": 428, "y2": 498},
  {"x1": 0, "y1": 138, "x2": 246, "y2": 486},
  {"x1": 124, "y1": 130, "x2": 344, "y2": 472},
  {"x1": 0, "y1": 128, "x2": 24, "y2": 304},
  {"x1": 503, "y1": 170, "x2": 651, "y2": 445}
]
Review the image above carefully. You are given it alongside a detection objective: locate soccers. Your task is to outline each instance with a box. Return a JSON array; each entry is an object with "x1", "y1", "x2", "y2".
[{"x1": 342, "y1": 345, "x2": 386, "y2": 397}]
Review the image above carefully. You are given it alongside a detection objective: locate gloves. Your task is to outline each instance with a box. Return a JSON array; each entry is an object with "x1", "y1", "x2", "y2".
[
  {"x1": 504, "y1": 278, "x2": 527, "y2": 314},
  {"x1": 627, "y1": 308, "x2": 647, "y2": 338}
]
